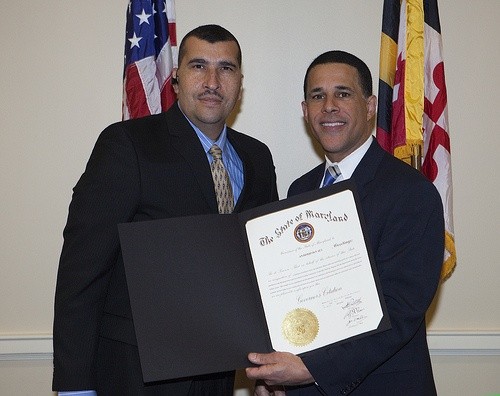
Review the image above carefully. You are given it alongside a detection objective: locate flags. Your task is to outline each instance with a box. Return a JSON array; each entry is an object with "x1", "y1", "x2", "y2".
[
  {"x1": 377, "y1": 0, "x2": 459, "y2": 331},
  {"x1": 124, "y1": 0, "x2": 181, "y2": 119}
]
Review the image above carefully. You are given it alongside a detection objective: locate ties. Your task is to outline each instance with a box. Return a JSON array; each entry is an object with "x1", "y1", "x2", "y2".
[
  {"x1": 209, "y1": 144, "x2": 236, "y2": 215},
  {"x1": 321, "y1": 165, "x2": 340, "y2": 188}
]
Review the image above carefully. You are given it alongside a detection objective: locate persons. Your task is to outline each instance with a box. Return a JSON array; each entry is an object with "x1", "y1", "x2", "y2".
[
  {"x1": 231, "y1": 49, "x2": 446, "y2": 396},
  {"x1": 43, "y1": 21, "x2": 278, "y2": 396}
]
